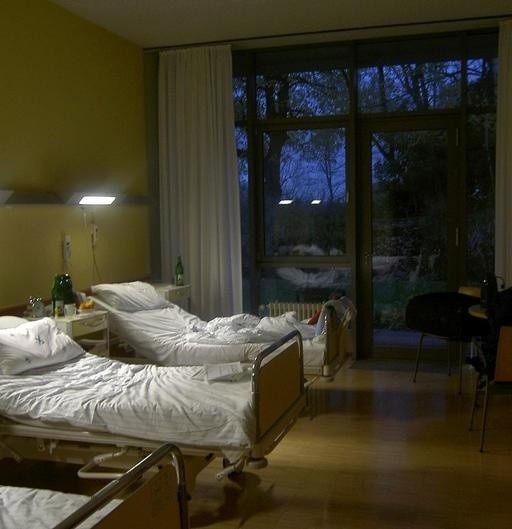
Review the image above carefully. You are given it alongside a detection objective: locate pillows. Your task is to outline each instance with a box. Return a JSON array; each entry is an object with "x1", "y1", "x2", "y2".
[
  {"x1": 0, "y1": 315, "x2": 87, "y2": 378},
  {"x1": 90, "y1": 278, "x2": 173, "y2": 314}
]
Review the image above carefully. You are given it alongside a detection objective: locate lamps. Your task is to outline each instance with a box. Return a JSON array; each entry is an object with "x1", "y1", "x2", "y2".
[{"x1": 67, "y1": 191, "x2": 118, "y2": 238}]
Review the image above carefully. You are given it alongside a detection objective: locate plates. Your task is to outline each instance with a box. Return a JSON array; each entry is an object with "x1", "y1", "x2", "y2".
[{"x1": 75, "y1": 308, "x2": 96, "y2": 314}]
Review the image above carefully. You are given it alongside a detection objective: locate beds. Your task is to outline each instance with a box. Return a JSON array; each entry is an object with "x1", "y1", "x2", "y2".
[
  {"x1": 0, "y1": 313, "x2": 313, "y2": 498},
  {"x1": 86, "y1": 292, "x2": 359, "y2": 410},
  {"x1": 0, "y1": 443, "x2": 191, "y2": 528}
]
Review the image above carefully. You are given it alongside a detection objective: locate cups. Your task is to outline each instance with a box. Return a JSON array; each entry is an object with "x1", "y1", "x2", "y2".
[{"x1": 62, "y1": 303, "x2": 77, "y2": 320}]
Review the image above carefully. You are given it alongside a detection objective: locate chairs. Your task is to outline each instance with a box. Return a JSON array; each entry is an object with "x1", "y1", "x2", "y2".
[
  {"x1": 457, "y1": 286, "x2": 488, "y2": 359},
  {"x1": 467, "y1": 325, "x2": 512, "y2": 452},
  {"x1": 403, "y1": 290, "x2": 482, "y2": 396}
]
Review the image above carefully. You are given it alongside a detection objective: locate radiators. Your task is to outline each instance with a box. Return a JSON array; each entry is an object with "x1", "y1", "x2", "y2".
[{"x1": 265, "y1": 301, "x2": 325, "y2": 324}]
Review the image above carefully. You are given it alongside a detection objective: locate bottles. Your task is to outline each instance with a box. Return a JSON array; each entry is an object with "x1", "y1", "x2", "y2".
[
  {"x1": 174, "y1": 255, "x2": 184, "y2": 286},
  {"x1": 50, "y1": 273, "x2": 73, "y2": 316},
  {"x1": 33, "y1": 297, "x2": 46, "y2": 318}
]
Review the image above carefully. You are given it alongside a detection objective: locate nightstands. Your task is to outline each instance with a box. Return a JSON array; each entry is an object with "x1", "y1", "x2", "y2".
[{"x1": 32, "y1": 310, "x2": 112, "y2": 359}]
